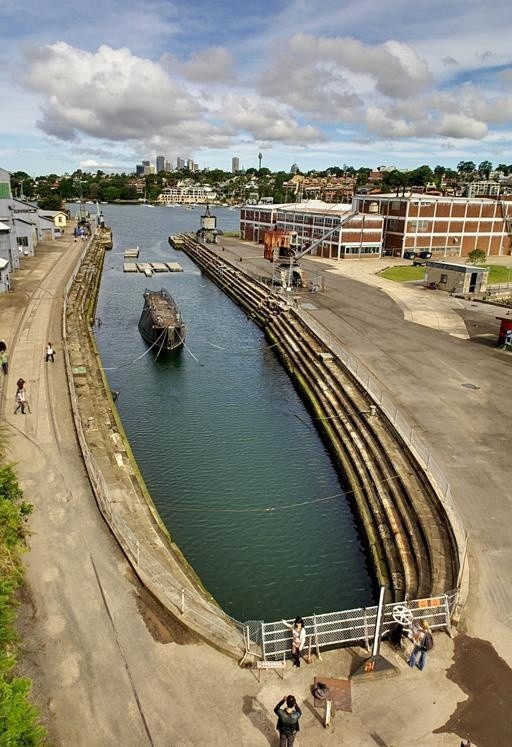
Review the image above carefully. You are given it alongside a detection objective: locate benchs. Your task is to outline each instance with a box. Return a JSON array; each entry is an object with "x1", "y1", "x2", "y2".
[{"x1": 249, "y1": 659, "x2": 286, "y2": 683}]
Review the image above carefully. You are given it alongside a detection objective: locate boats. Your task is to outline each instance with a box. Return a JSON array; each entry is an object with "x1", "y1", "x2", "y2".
[{"x1": 138, "y1": 287, "x2": 187, "y2": 351}]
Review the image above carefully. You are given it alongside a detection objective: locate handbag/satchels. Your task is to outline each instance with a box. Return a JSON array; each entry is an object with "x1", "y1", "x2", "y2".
[{"x1": 313, "y1": 683, "x2": 330, "y2": 699}]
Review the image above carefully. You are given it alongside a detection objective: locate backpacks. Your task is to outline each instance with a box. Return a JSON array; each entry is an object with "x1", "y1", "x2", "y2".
[{"x1": 421, "y1": 629, "x2": 433, "y2": 652}]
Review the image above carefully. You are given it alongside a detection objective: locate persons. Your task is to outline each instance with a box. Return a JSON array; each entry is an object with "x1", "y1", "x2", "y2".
[
  {"x1": 274, "y1": 695, "x2": 302, "y2": 747},
  {"x1": 45, "y1": 342, "x2": 56, "y2": 362},
  {"x1": 13, "y1": 378, "x2": 32, "y2": 415},
  {"x1": 406, "y1": 618, "x2": 434, "y2": 670},
  {"x1": 0, "y1": 350, "x2": 10, "y2": 376},
  {"x1": 0, "y1": 341, "x2": 6, "y2": 352},
  {"x1": 281, "y1": 616, "x2": 307, "y2": 667}
]
